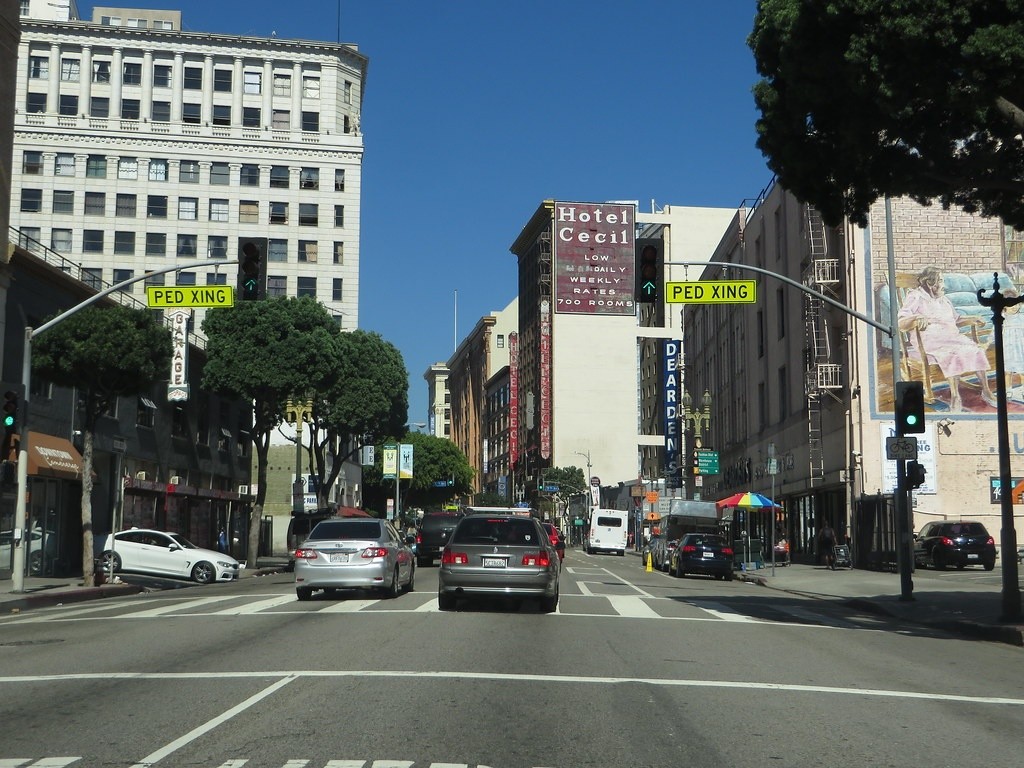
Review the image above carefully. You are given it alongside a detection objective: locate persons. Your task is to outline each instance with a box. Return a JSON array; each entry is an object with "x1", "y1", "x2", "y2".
[
  {"x1": 220, "y1": 527, "x2": 227, "y2": 552},
  {"x1": 775, "y1": 538, "x2": 787, "y2": 567},
  {"x1": 817, "y1": 520, "x2": 837, "y2": 569}
]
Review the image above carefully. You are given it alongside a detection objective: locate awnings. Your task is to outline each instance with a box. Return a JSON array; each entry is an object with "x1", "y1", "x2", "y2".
[{"x1": 9, "y1": 428, "x2": 97, "y2": 482}]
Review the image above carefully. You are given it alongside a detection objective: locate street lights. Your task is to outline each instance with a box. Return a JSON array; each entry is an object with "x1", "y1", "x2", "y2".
[
  {"x1": 683, "y1": 387, "x2": 713, "y2": 500},
  {"x1": 394, "y1": 421, "x2": 428, "y2": 530},
  {"x1": 286, "y1": 387, "x2": 316, "y2": 547},
  {"x1": 576, "y1": 449, "x2": 591, "y2": 519}
]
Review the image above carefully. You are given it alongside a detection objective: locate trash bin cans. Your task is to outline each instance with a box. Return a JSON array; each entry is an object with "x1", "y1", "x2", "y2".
[{"x1": 732, "y1": 539, "x2": 761, "y2": 566}]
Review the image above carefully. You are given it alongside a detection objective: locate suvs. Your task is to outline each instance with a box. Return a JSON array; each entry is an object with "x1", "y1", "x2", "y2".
[
  {"x1": 439, "y1": 506, "x2": 566, "y2": 614},
  {"x1": 396, "y1": 505, "x2": 466, "y2": 568}
]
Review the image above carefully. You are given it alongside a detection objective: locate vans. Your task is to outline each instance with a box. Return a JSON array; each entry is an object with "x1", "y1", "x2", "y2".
[{"x1": 585, "y1": 508, "x2": 629, "y2": 556}]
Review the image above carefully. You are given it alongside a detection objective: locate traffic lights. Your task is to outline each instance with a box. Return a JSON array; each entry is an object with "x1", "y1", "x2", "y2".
[
  {"x1": 634, "y1": 237, "x2": 666, "y2": 304},
  {"x1": 0, "y1": 382, "x2": 25, "y2": 436},
  {"x1": 896, "y1": 380, "x2": 925, "y2": 434},
  {"x1": 235, "y1": 236, "x2": 269, "y2": 302},
  {"x1": 537, "y1": 474, "x2": 545, "y2": 492}
]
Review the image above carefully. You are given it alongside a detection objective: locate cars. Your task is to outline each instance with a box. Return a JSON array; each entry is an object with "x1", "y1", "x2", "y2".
[
  {"x1": 540, "y1": 522, "x2": 567, "y2": 565},
  {"x1": 0, "y1": 524, "x2": 55, "y2": 578},
  {"x1": 286, "y1": 514, "x2": 327, "y2": 571},
  {"x1": 642, "y1": 533, "x2": 682, "y2": 572},
  {"x1": 668, "y1": 533, "x2": 735, "y2": 582},
  {"x1": 913, "y1": 520, "x2": 997, "y2": 572},
  {"x1": 293, "y1": 518, "x2": 418, "y2": 601},
  {"x1": 95, "y1": 526, "x2": 241, "y2": 585}
]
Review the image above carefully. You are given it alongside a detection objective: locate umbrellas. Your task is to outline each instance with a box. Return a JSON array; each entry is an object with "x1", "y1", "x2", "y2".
[{"x1": 715, "y1": 492, "x2": 784, "y2": 571}]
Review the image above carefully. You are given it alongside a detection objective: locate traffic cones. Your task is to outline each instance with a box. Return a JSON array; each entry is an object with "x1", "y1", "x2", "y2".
[{"x1": 645, "y1": 553, "x2": 653, "y2": 572}]
